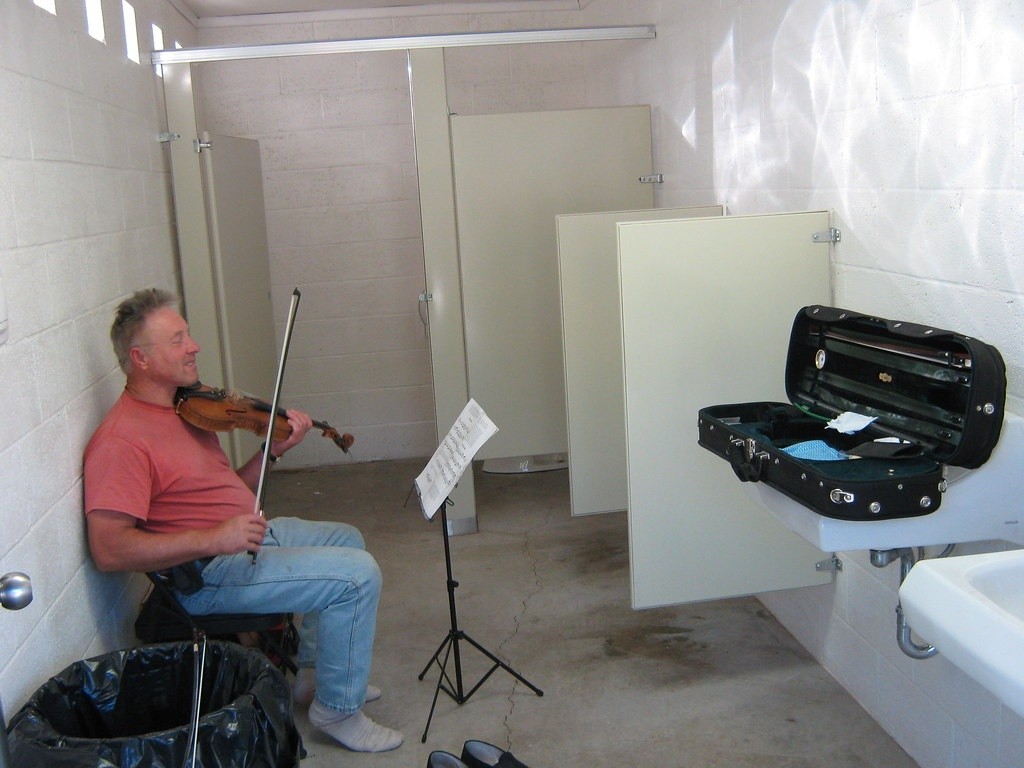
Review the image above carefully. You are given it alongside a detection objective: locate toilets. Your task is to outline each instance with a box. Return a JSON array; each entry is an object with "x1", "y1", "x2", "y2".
[{"x1": 480, "y1": 451, "x2": 568, "y2": 473}]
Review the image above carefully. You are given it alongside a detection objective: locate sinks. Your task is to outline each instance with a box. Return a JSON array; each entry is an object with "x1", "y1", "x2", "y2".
[
  {"x1": 744, "y1": 385, "x2": 1023, "y2": 552},
  {"x1": 898, "y1": 547, "x2": 1023, "y2": 719}
]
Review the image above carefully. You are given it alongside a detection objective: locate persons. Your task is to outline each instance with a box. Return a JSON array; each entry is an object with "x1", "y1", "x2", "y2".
[{"x1": 82, "y1": 288, "x2": 404, "y2": 752}]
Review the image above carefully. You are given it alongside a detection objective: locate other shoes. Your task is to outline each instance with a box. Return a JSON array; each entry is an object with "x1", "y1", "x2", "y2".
[
  {"x1": 426, "y1": 751, "x2": 469, "y2": 768},
  {"x1": 461, "y1": 739, "x2": 528, "y2": 768}
]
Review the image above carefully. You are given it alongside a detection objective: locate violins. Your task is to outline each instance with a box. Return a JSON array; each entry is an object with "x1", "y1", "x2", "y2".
[{"x1": 174, "y1": 381, "x2": 355, "y2": 454}]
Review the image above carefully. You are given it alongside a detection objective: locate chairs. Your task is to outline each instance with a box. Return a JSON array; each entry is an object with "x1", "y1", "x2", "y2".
[{"x1": 134, "y1": 571, "x2": 299, "y2": 677}]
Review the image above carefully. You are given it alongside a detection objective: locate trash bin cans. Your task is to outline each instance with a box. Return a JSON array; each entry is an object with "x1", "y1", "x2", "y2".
[{"x1": 8, "y1": 637, "x2": 284, "y2": 768}]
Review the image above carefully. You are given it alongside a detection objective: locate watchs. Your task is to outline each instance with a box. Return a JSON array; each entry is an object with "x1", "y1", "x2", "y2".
[{"x1": 260, "y1": 441, "x2": 281, "y2": 466}]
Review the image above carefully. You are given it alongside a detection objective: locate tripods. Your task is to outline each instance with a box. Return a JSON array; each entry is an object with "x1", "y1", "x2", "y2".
[{"x1": 414, "y1": 397, "x2": 543, "y2": 744}]
